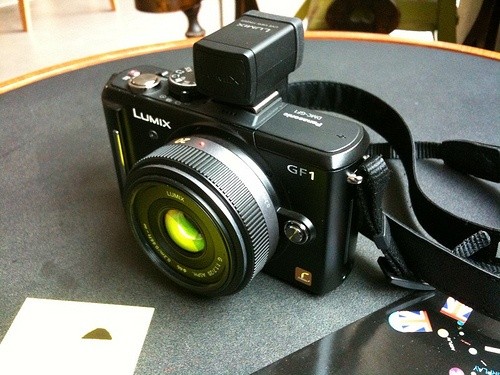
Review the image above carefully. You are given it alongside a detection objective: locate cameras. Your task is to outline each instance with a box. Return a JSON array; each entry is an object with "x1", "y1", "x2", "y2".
[{"x1": 101, "y1": 11, "x2": 371, "y2": 301}]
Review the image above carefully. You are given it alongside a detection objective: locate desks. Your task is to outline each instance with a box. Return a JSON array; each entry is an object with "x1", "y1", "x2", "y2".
[{"x1": 0, "y1": 30, "x2": 500, "y2": 375}]
